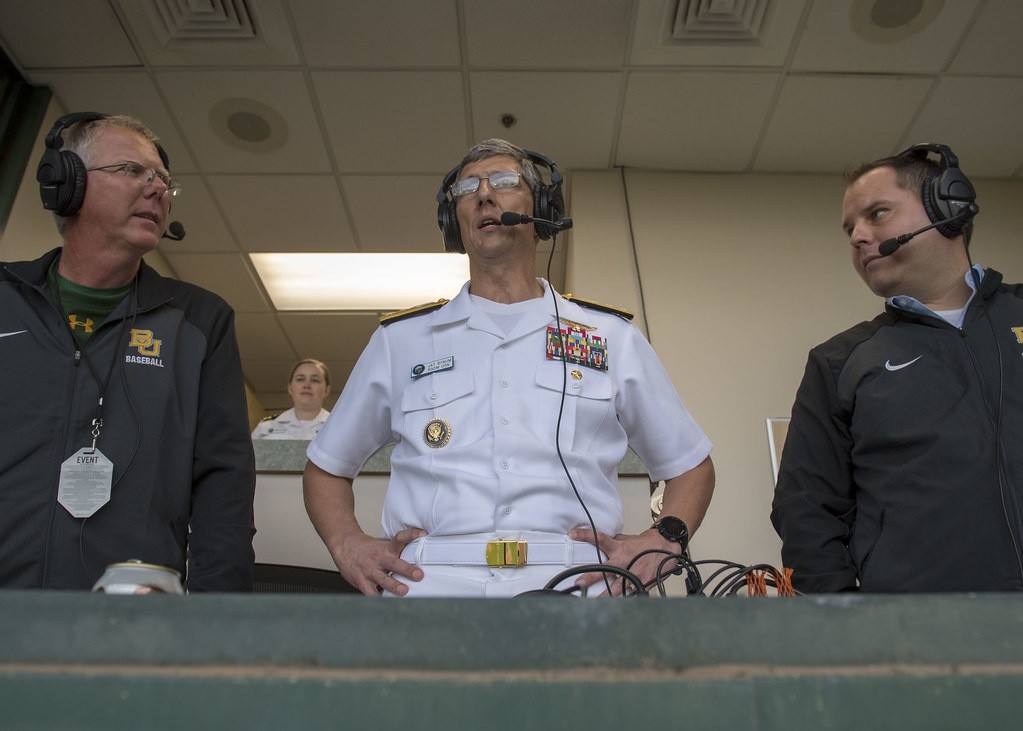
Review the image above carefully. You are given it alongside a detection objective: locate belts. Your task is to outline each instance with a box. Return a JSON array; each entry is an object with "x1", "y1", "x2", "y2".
[{"x1": 399, "y1": 540, "x2": 609, "y2": 565}]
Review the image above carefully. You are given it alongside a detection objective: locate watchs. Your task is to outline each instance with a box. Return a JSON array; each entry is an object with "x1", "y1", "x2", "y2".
[{"x1": 650, "y1": 515, "x2": 690, "y2": 577}]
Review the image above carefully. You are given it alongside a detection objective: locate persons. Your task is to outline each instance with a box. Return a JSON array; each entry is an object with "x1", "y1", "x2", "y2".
[
  {"x1": 1, "y1": 114, "x2": 258, "y2": 597},
  {"x1": 770, "y1": 143, "x2": 1023, "y2": 594},
  {"x1": 248, "y1": 360, "x2": 333, "y2": 441},
  {"x1": 307, "y1": 137, "x2": 716, "y2": 595}
]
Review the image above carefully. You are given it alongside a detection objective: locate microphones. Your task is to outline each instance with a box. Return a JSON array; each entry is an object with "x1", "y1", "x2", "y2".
[
  {"x1": 500, "y1": 211, "x2": 577, "y2": 234},
  {"x1": 162, "y1": 217, "x2": 183, "y2": 242},
  {"x1": 879, "y1": 212, "x2": 972, "y2": 257}
]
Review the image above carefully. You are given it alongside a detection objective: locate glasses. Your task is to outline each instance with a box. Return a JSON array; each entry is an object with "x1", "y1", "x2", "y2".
[
  {"x1": 84, "y1": 161, "x2": 182, "y2": 199},
  {"x1": 449, "y1": 172, "x2": 535, "y2": 202}
]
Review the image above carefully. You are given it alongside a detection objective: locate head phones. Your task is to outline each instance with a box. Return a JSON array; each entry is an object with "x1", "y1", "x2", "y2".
[
  {"x1": 435, "y1": 148, "x2": 567, "y2": 254},
  {"x1": 36, "y1": 112, "x2": 171, "y2": 220},
  {"x1": 901, "y1": 143, "x2": 978, "y2": 240}
]
